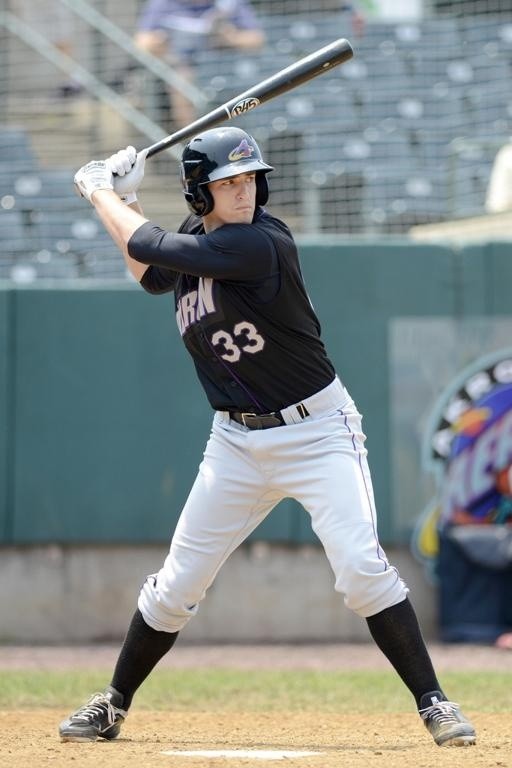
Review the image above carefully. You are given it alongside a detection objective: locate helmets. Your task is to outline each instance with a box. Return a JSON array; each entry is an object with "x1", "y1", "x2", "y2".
[{"x1": 182, "y1": 126, "x2": 274, "y2": 217}]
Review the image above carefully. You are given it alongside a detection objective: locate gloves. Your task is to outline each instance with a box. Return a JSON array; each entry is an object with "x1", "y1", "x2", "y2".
[{"x1": 74, "y1": 146, "x2": 149, "y2": 205}]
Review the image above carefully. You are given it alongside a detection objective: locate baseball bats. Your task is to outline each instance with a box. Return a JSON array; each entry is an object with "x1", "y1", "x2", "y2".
[{"x1": 73, "y1": 38, "x2": 354, "y2": 197}]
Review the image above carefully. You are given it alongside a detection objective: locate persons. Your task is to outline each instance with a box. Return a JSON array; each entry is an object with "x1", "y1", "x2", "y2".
[
  {"x1": 58, "y1": 126, "x2": 475, "y2": 747},
  {"x1": 134, "y1": 0, "x2": 266, "y2": 129}
]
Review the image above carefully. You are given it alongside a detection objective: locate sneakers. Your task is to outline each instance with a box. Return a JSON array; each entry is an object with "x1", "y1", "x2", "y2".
[
  {"x1": 419, "y1": 690, "x2": 475, "y2": 746},
  {"x1": 59, "y1": 686, "x2": 126, "y2": 742}
]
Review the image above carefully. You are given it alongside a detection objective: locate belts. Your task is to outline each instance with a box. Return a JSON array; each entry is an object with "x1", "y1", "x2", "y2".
[{"x1": 229, "y1": 403, "x2": 310, "y2": 429}]
